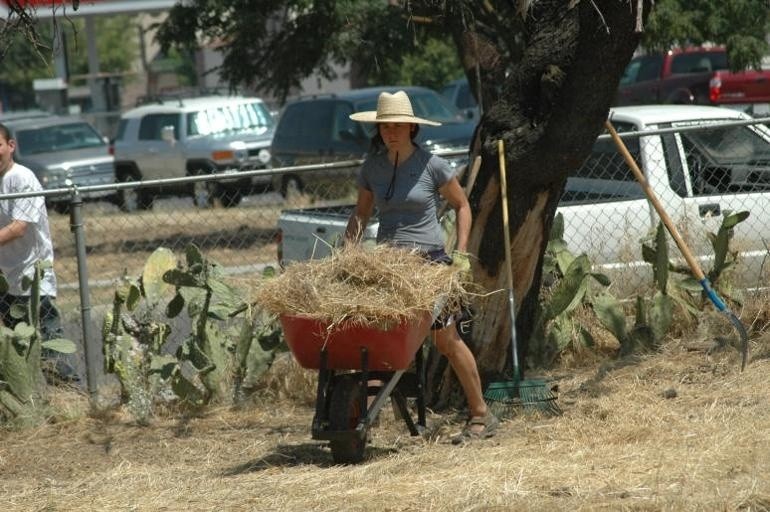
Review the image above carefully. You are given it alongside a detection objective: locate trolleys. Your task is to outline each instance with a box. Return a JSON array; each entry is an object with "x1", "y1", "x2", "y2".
[{"x1": 279, "y1": 232, "x2": 473, "y2": 466}]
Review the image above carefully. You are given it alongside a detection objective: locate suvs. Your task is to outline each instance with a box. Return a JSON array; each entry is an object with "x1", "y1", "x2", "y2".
[
  {"x1": 111, "y1": 90, "x2": 285, "y2": 216},
  {"x1": 2, "y1": 110, "x2": 121, "y2": 217}
]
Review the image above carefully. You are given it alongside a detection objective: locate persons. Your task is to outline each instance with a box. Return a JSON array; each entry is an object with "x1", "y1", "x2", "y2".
[
  {"x1": 0, "y1": 122, "x2": 59, "y2": 333},
  {"x1": 340, "y1": 121, "x2": 500, "y2": 447}
]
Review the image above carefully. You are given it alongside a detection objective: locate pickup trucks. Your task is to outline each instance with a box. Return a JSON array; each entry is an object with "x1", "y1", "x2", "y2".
[
  {"x1": 615, "y1": 42, "x2": 770, "y2": 103},
  {"x1": 279, "y1": 102, "x2": 770, "y2": 339}
]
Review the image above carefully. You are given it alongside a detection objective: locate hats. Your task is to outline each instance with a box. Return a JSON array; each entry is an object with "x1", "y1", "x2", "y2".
[{"x1": 347, "y1": 89, "x2": 445, "y2": 128}]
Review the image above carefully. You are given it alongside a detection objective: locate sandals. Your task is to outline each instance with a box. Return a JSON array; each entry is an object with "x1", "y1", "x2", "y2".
[{"x1": 451, "y1": 407, "x2": 499, "y2": 446}]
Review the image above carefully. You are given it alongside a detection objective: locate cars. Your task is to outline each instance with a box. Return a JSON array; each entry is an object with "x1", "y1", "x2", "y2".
[
  {"x1": 440, "y1": 79, "x2": 483, "y2": 122},
  {"x1": 267, "y1": 85, "x2": 482, "y2": 207}
]
